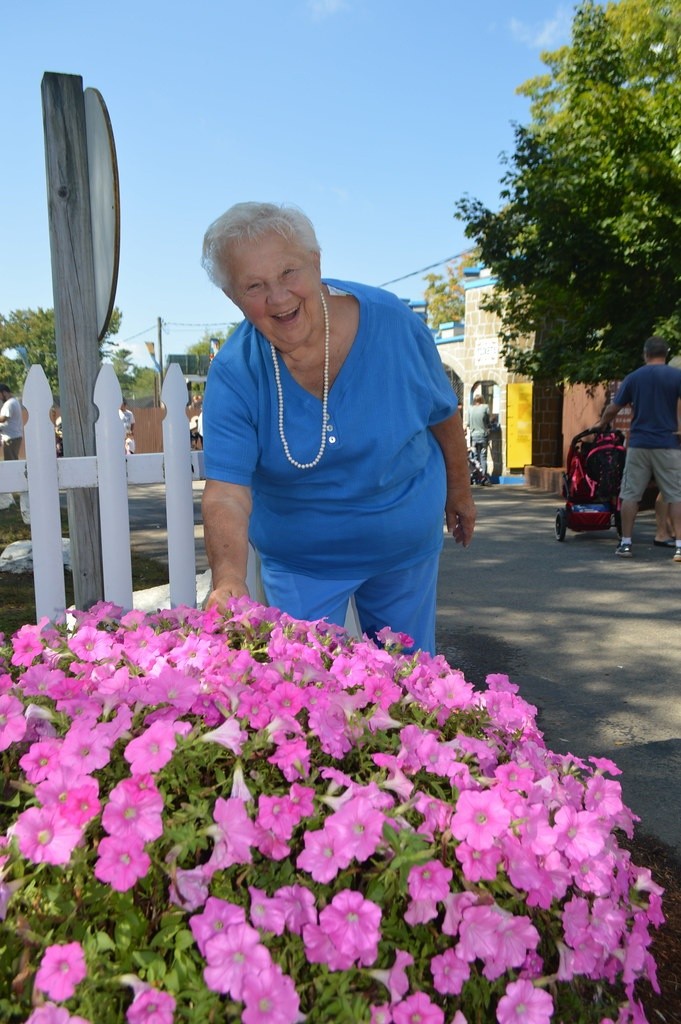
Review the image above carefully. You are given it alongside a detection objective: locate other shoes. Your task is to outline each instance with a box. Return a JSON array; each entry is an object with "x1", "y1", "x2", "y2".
[
  {"x1": 673, "y1": 547, "x2": 680, "y2": 561},
  {"x1": 614, "y1": 543, "x2": 632, "y2": 557}
]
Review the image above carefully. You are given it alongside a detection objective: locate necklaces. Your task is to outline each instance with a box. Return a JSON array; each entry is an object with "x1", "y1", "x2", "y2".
[{"x1": 270, "y1": 289, "x2": 330, "y2": 469}]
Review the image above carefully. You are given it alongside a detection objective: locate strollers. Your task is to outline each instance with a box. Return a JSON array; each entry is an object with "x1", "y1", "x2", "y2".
[{"x1": 554, "y1": 427, "x2": 627, "y2": 542}]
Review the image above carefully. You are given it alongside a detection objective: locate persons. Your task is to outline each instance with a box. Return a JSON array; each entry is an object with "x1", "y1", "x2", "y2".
[
  {"x1": 201, "y1": 200, "x2": 478, "y2": 660},
  {"x1": 56, "y1": 427, "x2": 63, "y2": 457},
  {"x1": 654, "y1": 356, "x2": 681, "y2": 548},
  {"x1": 119, "y1": 396, "x2": 136, "y2": 439},
  {"x1": 190, "y1": 414, "x2": 201, "y2": 449},
  {"x1": 0, "y1": 383, "x2": 23, "y2": 509},
  {"x1": 589, "y1": 336, "x2": 681, "y2": 561},
  {"x1": 125, "y1": 430, "x2": 136, "y2": 455},
  {"x1": 466, "y1": 394, "x2": 495, "y2": 485}
]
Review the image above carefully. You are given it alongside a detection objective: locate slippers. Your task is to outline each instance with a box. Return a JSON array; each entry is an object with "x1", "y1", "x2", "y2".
[{"x1": 653, "y1": 538, "x2": 675, "y2": 548}]
